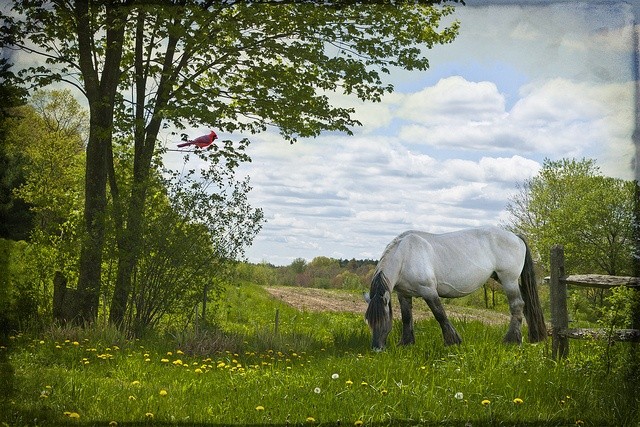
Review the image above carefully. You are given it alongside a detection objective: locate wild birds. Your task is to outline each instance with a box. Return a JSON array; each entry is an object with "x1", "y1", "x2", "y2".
[{"x1": 177, "y1": 129, "x2": 219, "y2": 149}]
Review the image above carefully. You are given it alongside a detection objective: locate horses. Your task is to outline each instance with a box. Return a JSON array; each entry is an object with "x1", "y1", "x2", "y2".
[{"x1": 363, "y1": 226, "x2": 548, "y2": 352}]
